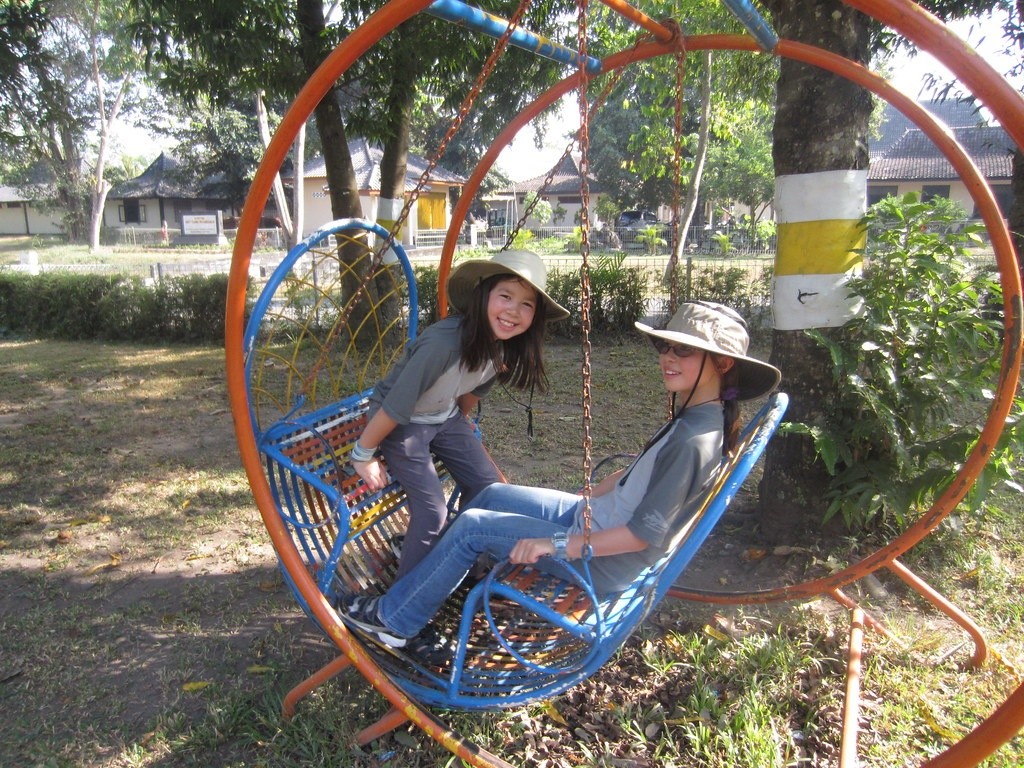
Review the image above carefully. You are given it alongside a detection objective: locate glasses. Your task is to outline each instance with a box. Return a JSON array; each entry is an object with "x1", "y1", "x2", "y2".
[{"x1": 653, "y1": 340, "x2": 705, "y2": 358}]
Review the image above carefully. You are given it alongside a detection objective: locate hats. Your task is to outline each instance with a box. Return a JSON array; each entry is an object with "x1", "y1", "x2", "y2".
[
  {"x1": 446, "y1": 248, "x2": 570, "y2": 323},
  {"x1": 634, "y1": 300, "x2": 781, "y2": 403}
]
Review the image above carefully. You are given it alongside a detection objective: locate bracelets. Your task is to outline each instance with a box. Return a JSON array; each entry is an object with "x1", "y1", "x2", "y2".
[{"x1": 351, "y1": 441, "x2": 376, "y2": 463}]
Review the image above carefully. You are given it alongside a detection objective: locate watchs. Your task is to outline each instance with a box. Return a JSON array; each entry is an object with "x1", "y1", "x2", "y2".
[{"x1": 551, "y1": 531, "x2": 569, "y2": 559}]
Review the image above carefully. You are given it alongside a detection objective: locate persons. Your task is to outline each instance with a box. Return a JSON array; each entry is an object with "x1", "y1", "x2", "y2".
[
  {"x1": 349, "y1": 249, "x2": 570, "y2": 666},
  {"x1": 336, "y1": 301, "x2": 782, "y2": 647}
]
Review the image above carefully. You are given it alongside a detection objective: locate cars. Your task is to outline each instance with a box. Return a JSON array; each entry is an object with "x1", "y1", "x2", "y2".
[{"x1": 620, "y1": 210, "x2": 658, "y2": 228}]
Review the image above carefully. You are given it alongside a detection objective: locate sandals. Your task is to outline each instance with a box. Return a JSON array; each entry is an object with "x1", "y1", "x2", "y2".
[
  {"x1": 461, "y1": 563, "x2": 495, "y2": 589},
  {"x1": 406, "y1": 623, "x2": 456, "y2": 665}
]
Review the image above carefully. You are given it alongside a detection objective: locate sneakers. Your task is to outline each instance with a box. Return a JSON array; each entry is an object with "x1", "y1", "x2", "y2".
[
  {"x1": 337, "y1": 591, "x2": 406, "y2": 647},
  {"x1": 390, "y1": 533, "x2": 491, "y2": 591}
]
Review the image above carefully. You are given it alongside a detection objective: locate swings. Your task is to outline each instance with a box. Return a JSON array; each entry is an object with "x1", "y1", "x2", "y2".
[{"x1": 236, "y1": 0, "x2": 792, "y2": 714}]
